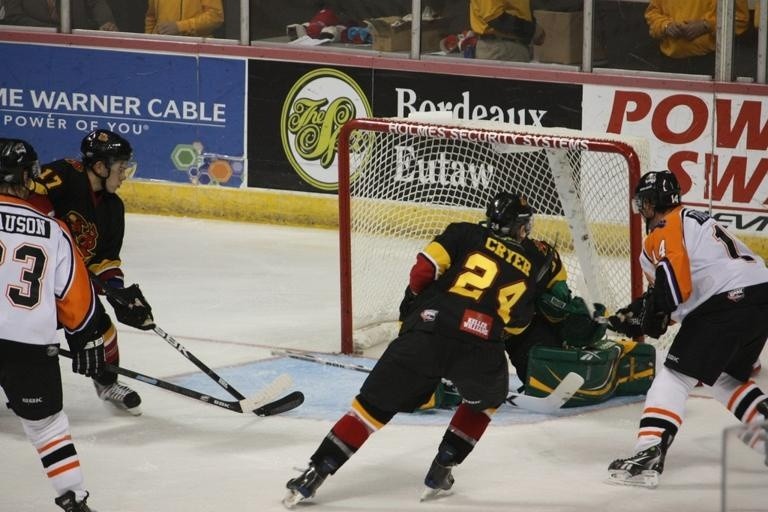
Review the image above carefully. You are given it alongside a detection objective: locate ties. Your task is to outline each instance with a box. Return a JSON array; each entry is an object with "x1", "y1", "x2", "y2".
[{"x1": 47, "y1": 1, "x2": 59, "y2": 27}]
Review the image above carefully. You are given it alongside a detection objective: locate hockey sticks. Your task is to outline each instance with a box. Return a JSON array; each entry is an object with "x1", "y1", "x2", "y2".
[
  {"x1": 89, "y1": 270, "x2": 304, "y2": 416},
  {"x1": 60, "y1": 348, "x2": 293, "y2": 414},
  {"x1": 270, "y1": 347, "x2": 583, "y2": 415}
]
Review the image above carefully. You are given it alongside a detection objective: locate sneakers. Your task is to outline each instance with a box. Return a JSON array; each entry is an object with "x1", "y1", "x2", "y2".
[
  {"x1": 609, "y1": 445, "x2": 665, "y2": 476},
  {"x1": 287, "y1": 463, "x2": 330, "y2": 497},
  {"x1": 424, "y1": 453, "x2": 455, "y2": 490},
  {"x1": 94, "y1": 379, "x2": 141, "y2": 409},
  {"x1": 54, "y1": 489, "x2": 94, "y2": 511}
]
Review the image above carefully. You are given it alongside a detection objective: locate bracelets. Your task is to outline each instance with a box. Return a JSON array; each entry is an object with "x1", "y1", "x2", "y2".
[{"x1": 703, "y1": 18, "x2": 711, "y2": 34}]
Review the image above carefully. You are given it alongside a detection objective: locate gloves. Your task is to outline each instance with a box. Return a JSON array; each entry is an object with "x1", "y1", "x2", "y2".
[
  {"x1": 107, "y1": 284, "x2": 156, "y2": 330},
  {"x1": 441, "y1": 31, "x2": 476, "y2": 60},
  {"x1": 307, "y1": 6, "x2": 392, "y2": 44},
  {"x1": 72, "y1": 330, "x2": 107, "y2": 379}
]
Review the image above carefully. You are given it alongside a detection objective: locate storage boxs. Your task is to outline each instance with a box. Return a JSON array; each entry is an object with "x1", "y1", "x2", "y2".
[
  {"x1": 362, "y1": 15, "x2": 410, "y2": 53},
  {"x1": 420, "y1": 19, "x2": 452, "y2": 52},
  {"x1": 534, "y1": 6, "x2": 606, "y2": 67}
]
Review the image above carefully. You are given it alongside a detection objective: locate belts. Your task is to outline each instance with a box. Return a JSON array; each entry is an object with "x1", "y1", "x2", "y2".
[{"x1": 477, "y1": 33, "x2": 495, "y2": 40}]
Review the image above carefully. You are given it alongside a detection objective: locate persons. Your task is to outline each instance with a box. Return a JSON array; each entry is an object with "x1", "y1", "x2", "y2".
[
  {"x1": 1, "y1": 138, "x2": 108, "y2": 512},
  {"x1": 466, "y1": 0, "x2": 546, "y2": 63},
  {"x1": 285, "y1": 191, "x2": 554, "y2": 504},
  {"x1": 642, "y1": 0, "x2": 751, "y2": 76},
  {"x1": 21, "y1": 129, "x2": 158, "y2": 410},
  {"x1": 0, "y1": 1, "x2": 122, "y2": 33},
  {"x1": 604, "y1": 170, "x2": 768, "y2": 478},
  {"x1": 144, "y1": 1, "x2": 225, "y2": 39},
  {"x1": 406, "y1": 195, "x2": 657, "y2": 416}
]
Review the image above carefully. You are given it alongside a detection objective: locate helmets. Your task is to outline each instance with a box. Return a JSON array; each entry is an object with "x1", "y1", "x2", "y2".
[
  {"x1": 486, "y1": 191, "x2": 531, "y2": 235},
  {"x1": 0, "y1": 138, "x2": 37, "y2": 184},
  {"x1": 80, "y1": 129, "x2": 132, "y2": 164},
  {"x1": 636, "y1": 170, "x2": 681, "y2": 211}
]
